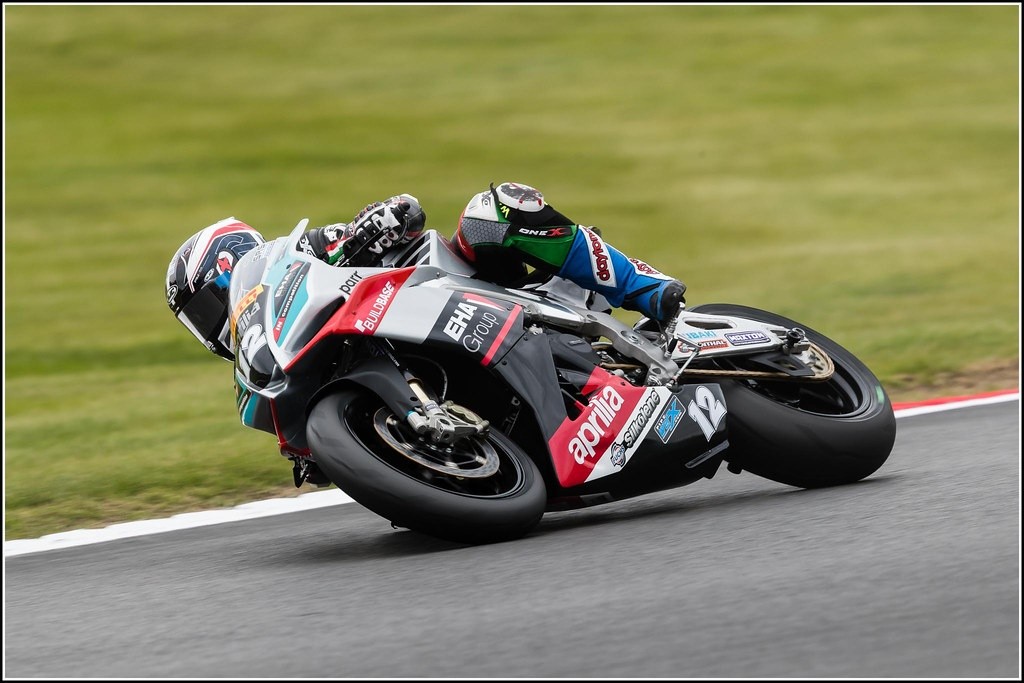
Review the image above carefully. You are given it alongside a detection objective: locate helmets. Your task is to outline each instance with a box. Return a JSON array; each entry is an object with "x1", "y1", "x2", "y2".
[{"x1": 164, "y1": 216, "x2": 270, "y2": 363}]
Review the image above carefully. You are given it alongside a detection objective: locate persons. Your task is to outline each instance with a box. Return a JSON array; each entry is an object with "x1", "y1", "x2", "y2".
[{"x1": 165, "y1": 181, "x2": 686, "y2": 487}]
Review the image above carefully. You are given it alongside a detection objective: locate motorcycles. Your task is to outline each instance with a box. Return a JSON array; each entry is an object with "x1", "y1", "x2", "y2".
[{"x1": 227, "y1": 201, "x2": 897, "y2": 545}]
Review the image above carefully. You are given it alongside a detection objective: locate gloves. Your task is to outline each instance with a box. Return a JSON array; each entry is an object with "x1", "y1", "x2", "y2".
[{"x1": 345, "y1": 200, "x2": 411, "y2": 254}]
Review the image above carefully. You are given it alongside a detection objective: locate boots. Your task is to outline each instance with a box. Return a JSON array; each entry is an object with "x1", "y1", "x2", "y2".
[{"x1": 558, "y1": 224, "x2": 688, "y2": 325}]
